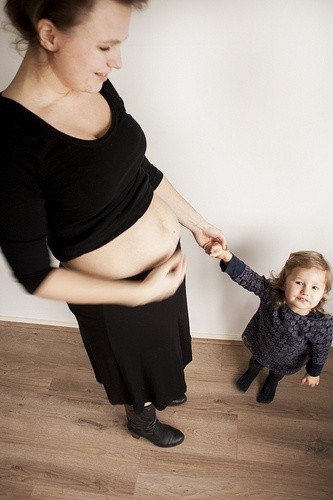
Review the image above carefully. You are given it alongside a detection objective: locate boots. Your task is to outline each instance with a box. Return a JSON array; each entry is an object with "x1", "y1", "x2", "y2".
[
  {"x1": 169, "y1": 395, "x2": 186, "y2": 405},
  {"x1": 124, "y1": 402, "x2": 184, "y2": 448}
]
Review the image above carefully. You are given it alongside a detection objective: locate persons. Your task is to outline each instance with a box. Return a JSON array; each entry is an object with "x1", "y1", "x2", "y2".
[
  {"x1": 0, "y1": 0, "x2": 229, "y2": 447},
  {"x1": 204, "y1": 240, "x2": 333, "y2": 403}
]
